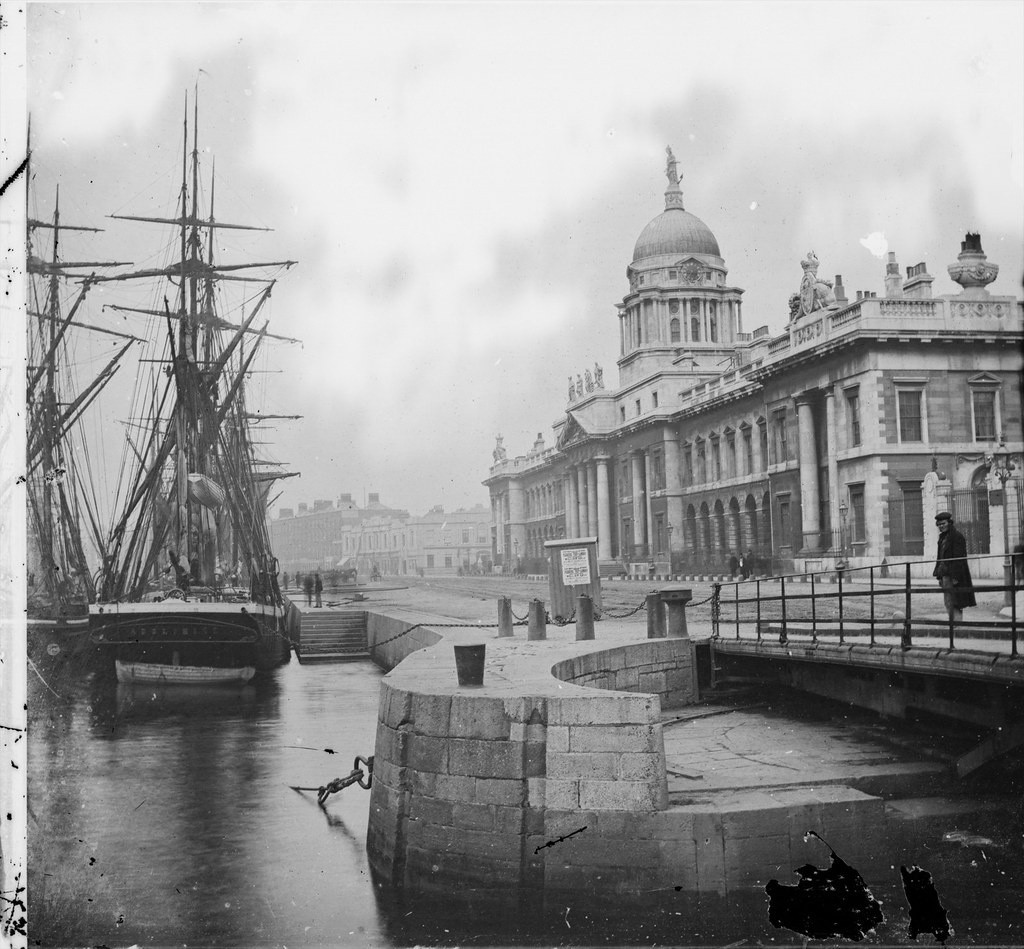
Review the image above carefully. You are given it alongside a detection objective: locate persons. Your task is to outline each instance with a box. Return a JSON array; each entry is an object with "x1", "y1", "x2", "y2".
[
  {"x1": 933, "y1": 512, "x2": 977, "y2": 628},
  {"x1": 664, "y1": 147, "x2": 678, "y2": 184},
  {"x1": 794, "y1": 252, "x2": 833, "y2": 324},
  {"x1": 730, "y1": 549, "x2": 754, "y2": 580},
  {"x1": 373, "y1": 566, "x2": 377, "y2": 581},
  {"x1": 495, "y1": 433, "x2": 504, "y2": 448},
  {"x1": 568, "y1": 362, "x2": 605, "y2": 402},
  {"x1": 283, "y1": 568, "x2": 323, "y2": 609}
]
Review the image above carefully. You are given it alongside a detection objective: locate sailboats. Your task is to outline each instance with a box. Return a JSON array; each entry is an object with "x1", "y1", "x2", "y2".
[
  {"x1": 25, "y1": 186, "x2": 152, "y2": 667},
  {"x1": 80, "y1": 71, "x2": 299, "y2": 687}
]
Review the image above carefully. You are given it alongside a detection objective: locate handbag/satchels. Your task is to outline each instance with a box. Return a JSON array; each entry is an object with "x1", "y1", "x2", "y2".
[{"x1": 949, "y1": 585, "x2": 977, "y2": 609}]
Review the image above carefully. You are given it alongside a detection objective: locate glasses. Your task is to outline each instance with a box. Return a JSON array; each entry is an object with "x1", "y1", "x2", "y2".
[{"x1": 936, "y1": 522, "x2": 949, "y2": 527}]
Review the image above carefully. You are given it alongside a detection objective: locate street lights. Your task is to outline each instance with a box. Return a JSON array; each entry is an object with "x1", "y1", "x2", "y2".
[
  {"x1": 993, "y1": 437, "x2": 1017, "y2": 607},
  {"x1": 838, "y1": 498, "x2": 852, "y2": 584},
  {"x1": 666, "y1": 521, "x2": 674, "y2": 582},
  {"x1": 512, "y1": 538, "x2": 520, "y2": 580}
]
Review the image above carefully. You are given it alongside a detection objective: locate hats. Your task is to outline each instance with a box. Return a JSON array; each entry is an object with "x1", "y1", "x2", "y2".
[{"x1": 935, "y1": 512, "x2": 952, "y2": 521}]
